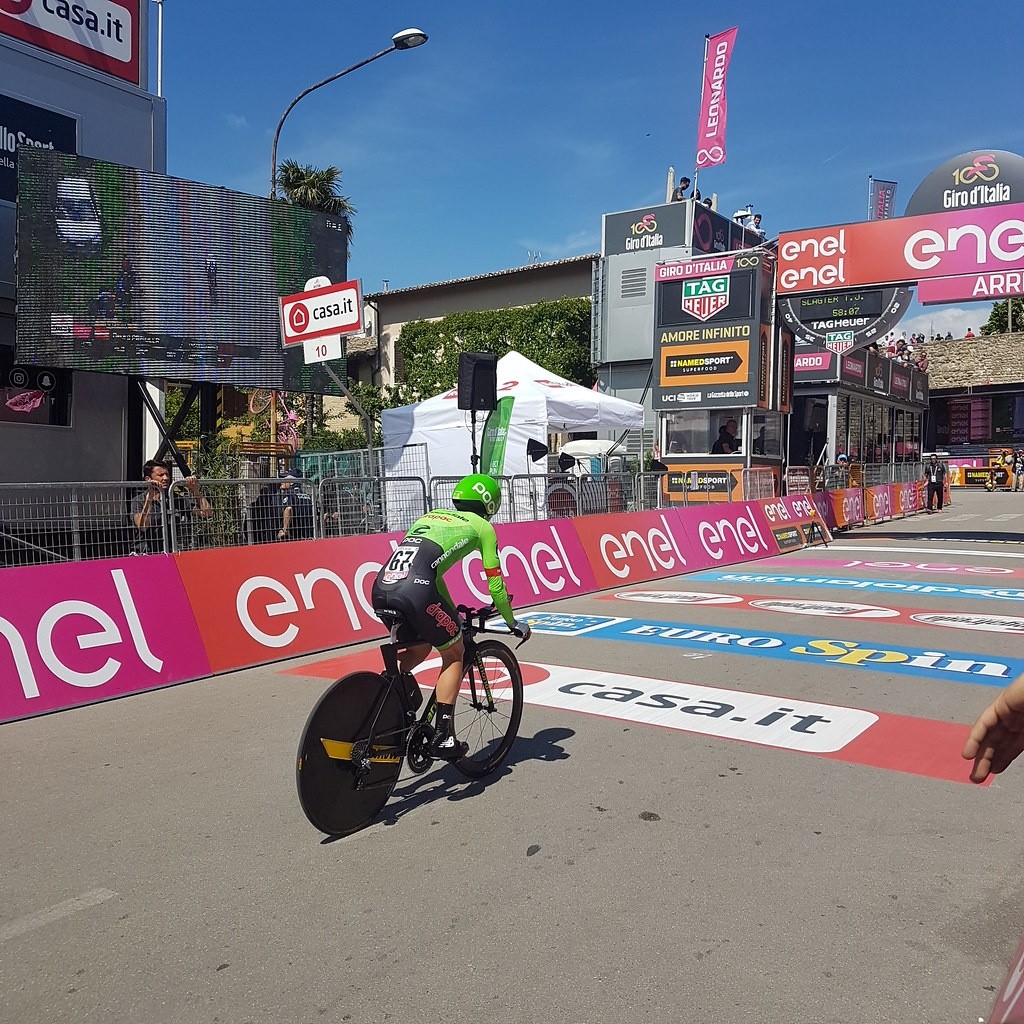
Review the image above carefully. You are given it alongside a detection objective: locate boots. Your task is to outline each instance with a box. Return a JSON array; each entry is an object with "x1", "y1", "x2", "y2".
[{"x1": 429, "y1": 702, "x2": 469, "y2": 759}]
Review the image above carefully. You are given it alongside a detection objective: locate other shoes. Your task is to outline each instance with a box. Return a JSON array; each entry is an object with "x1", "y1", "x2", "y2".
[
  {"x1": 938, "y1": 509, "x2": 942, "y2": 512},
  {"x1": 926, "y1": 509, "x2": 932, "y2": 515}
]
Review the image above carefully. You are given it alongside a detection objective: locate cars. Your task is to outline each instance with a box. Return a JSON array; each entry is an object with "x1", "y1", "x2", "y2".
[
  {"x1": 52, "y1": 176, "x2": 106, "y2": 259},
  {"x1": 863, "y1": 434, "x2": 918, "y2": 461}
]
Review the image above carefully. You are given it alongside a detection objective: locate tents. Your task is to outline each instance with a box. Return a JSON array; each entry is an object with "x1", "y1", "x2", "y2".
[{"x1": 379, "y1": 350, "x2": 646, "y2": 533}]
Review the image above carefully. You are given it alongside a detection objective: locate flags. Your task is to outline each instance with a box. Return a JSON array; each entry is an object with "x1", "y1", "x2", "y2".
[
  {"x1": 871, "y1": 177, "x2": 898, "y2": 221},
  {"x1": 694, "y1": 26, "x2": 739, "y2": 170}
]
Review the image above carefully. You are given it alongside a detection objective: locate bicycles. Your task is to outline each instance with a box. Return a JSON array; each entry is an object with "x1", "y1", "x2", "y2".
[
  {"x1": 249, "y1": 388, "x2": 300, "y2": 453},
  {"x1": 294, "y1": 595, "x2": 534, "y2": 835},
  {"x1": 207, "y1": 269, "x2": 217, "y2": 287}
]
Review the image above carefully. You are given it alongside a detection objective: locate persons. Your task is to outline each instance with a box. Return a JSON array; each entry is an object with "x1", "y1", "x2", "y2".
[
  {"x1": 711, "y1": 418, "x2": 738, "y2": 454},
  {"x1": 702, "y1": 197, "x2": 712, "y2": 208},
  {"x1": 242, "y1": 468, "x2": 382, "y2": 544},
  {"x1": 370, "y1": 473, "x2": 531, "y2": 759},
  {"x1": 744, "y1": 213, "x2": 766, "y2": 236},
  {"x1": 752, "y1": 425, "x2": 775, "y2": 455},
  {"x1": 128, "y1": 460, "x2": 212, "y2": 556},
  {"x1": 689, "y1": 188, "x2": 701, "y2": 201},
  {"x1": 731, "y1": 209, "x2": 743, "y2": 225},
  {"x1": 924, "y1": 454, "x2": 948, "y2": 515},
  {"x1": 828, "y1": 453, "x2": 858, "y2": 530},
  {"x1": 1003, "y1": 451, "x2": 1024, "y2": 491},
  {"x1": 670, "y1": 176, "x2": 690, "y2": 202},
  {"x1": 879, "y1": 327, "x2": 975, "y2": 373},
  {"x1": 984, "y1": 468, "x2": 998, "y2": 491}
]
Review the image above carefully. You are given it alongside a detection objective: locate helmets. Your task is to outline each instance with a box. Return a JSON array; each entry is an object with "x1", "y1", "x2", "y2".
[{"x1": 452, "y1": 474, "x2": 501, "y2": 515}]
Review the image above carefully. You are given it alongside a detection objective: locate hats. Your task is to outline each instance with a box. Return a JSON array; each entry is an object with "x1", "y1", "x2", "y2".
[
  {"x1": 279, "y1": 469, "x2": 303, "y2": 478},
  {"x1": 907, "y1": 346, "x2": 914, "y2": 352},
  {"x1": 838, "y1": 454, "x2": 847, "y2": 460},
  {"x1": 1018, "y1": 449, "x2": 1024, "y2": 453}
]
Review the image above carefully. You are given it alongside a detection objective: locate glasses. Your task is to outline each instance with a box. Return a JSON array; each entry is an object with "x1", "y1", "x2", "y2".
[
  {"x1": 930, "y1": 458, "x2": 936, "y2": 460},
  {"x1": 685, "y1": 183, "x2": 689, "y2": 186}
]
